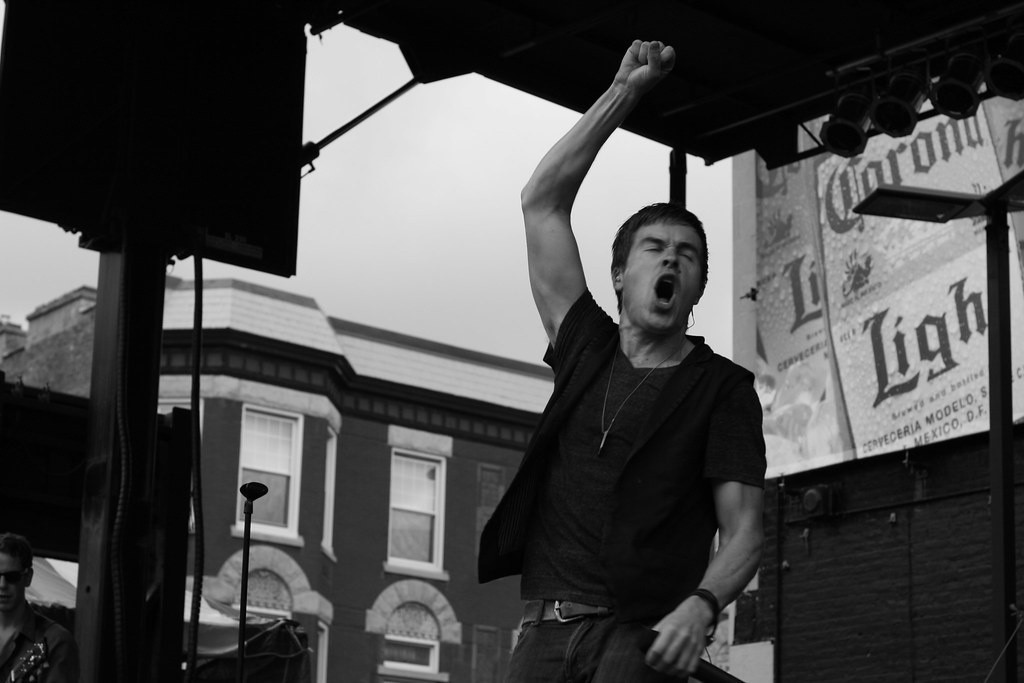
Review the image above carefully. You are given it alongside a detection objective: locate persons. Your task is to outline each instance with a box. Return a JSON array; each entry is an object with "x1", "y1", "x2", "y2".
[
  {"x1": 478, "y1": 41, "x2": 769, "y2": 683},
  {"x1": 0, "y1": 533, "x2": 80, "y2": 683}
]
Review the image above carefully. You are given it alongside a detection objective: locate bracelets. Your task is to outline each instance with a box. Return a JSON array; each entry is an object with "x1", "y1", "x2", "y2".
[{"x1": 692, "y1": 588, "x2": 719, "y2": 625}]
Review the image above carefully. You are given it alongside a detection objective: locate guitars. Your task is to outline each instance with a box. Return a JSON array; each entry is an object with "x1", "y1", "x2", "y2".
[{"x1": 2, "y1": 636, "x2": 51, "y2": 682}]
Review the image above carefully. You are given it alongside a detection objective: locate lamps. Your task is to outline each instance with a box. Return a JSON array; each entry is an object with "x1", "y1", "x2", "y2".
[
  {"x1": 818, "y1": 66, "x2": 878, "y2": 159},
  {"x1": 869, "y1": 47, "x2": 933, "y2": 137},
  {"x1": 931, "y1": 25, "x2": 991, "y2": 121},
  {"x1": 985, "y1": 14, "x2": 1024, "y2": 101}
]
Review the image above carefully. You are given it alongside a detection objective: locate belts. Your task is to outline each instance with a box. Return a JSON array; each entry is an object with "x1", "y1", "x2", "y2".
[{"x1": 522, "y1": 598, "x2": 616, "y2": 626}]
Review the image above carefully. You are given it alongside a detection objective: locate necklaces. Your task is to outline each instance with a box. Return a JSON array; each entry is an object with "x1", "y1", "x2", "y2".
[{"x1": 599, "y1": 340, "x2": 684, "y2": 452}]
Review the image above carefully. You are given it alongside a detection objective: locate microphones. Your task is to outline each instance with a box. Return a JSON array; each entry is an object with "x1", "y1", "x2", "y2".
[{"x1": 633, "y1": 627, "x2": 748, "y2": 683}]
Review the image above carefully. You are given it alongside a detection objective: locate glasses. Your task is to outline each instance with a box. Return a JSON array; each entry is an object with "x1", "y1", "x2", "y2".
[{"x1": 0, "y1": 567, "x2": 29, "y2": 585}]
